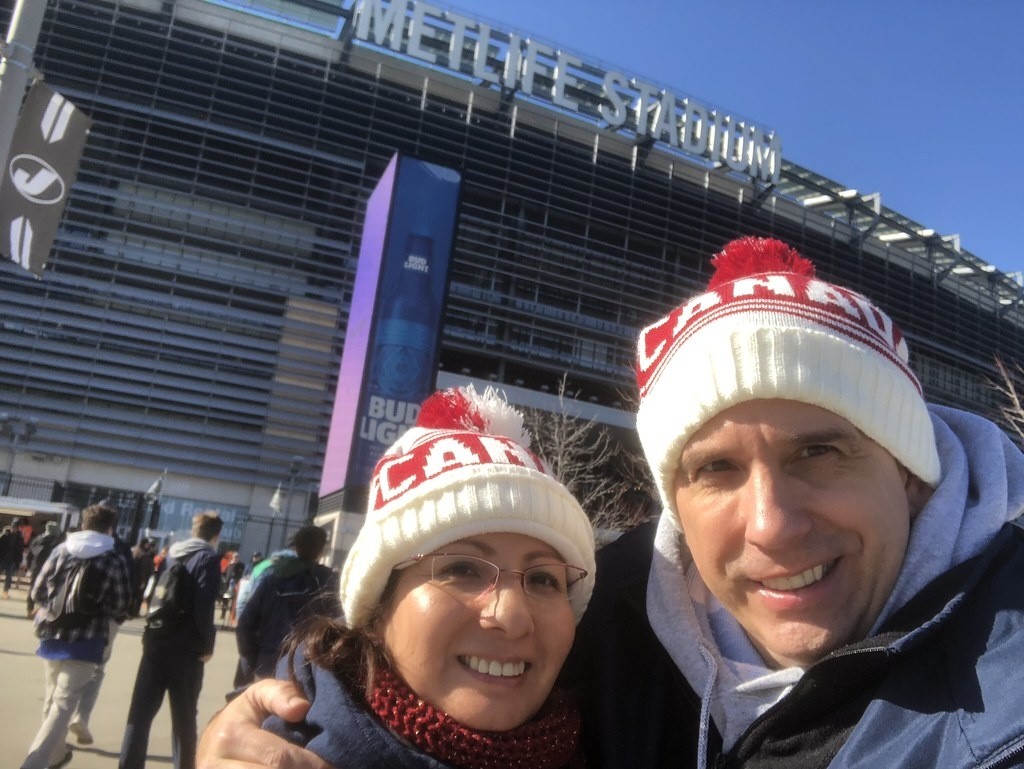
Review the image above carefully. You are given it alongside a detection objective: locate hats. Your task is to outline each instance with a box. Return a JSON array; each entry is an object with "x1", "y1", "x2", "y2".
[
  {"x1": 253, "y1": 552, "x2": 261, "y2": 557},
  {"x1": 46, "y1": 521, "x2": 60, "y2": 536},
  {"x1": 635, "y1": 237, "x2": 942, "y2": 532},
  {"x1": 12, "y1": 519, "x2": 21, "y2": 528},
  {"x1": 340, "y1": 384, "x2": 596, "y2": 633}
]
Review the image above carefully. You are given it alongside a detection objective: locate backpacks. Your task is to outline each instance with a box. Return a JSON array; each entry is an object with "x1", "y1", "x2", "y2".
[
  {"x1": 146, "y1": 552, "x2": 198, "y2": 638},
  {"x1": 42, "y1": 550, "x2": 115, "y2": 628}
]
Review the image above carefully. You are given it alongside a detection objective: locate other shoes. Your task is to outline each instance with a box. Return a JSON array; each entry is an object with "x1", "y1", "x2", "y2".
[
  {"x1": 2, "y1": 592, "x2": 9, "y2": 599},
  {"x1": 26, "y1": 611, "x2": 36, "y2": 619},
  {"x1": 49, "y1": 751, "x2": 72, "y2": 769},
  {"x1": 69, "y1": 715, "x2": 93, "y2": 744}
]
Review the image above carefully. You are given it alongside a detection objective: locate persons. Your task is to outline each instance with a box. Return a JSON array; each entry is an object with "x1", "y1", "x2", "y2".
[
  {"x1": 15, "y1": 501, "x2": 134, "y2": 769},
  {"x1": 118, "y1": 510, "x2": 224, "y2": 769},
  {"x1": 21, "y1": 520, "x2": 267, "y2": 630},
  {"x1": 0, "y1": 516, "x2": 33, "y2": 600},
  {"x1": 233, "y1": 525, "x2": 341, "y2": 688},
  {"x1": 68, "y1": 510, "x2": 134, "y2": 745},
  {"x1": 194, "y1": 232, "x2": 1024, "y2": 769},
  {"x1": 259, "y1": 381, "x2": 603, "y2": 769}
]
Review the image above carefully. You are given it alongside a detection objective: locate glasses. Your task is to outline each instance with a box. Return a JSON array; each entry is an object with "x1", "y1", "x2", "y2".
[{"x1": 393, "y1": 553, "x2": 589, "y2": 602}]
